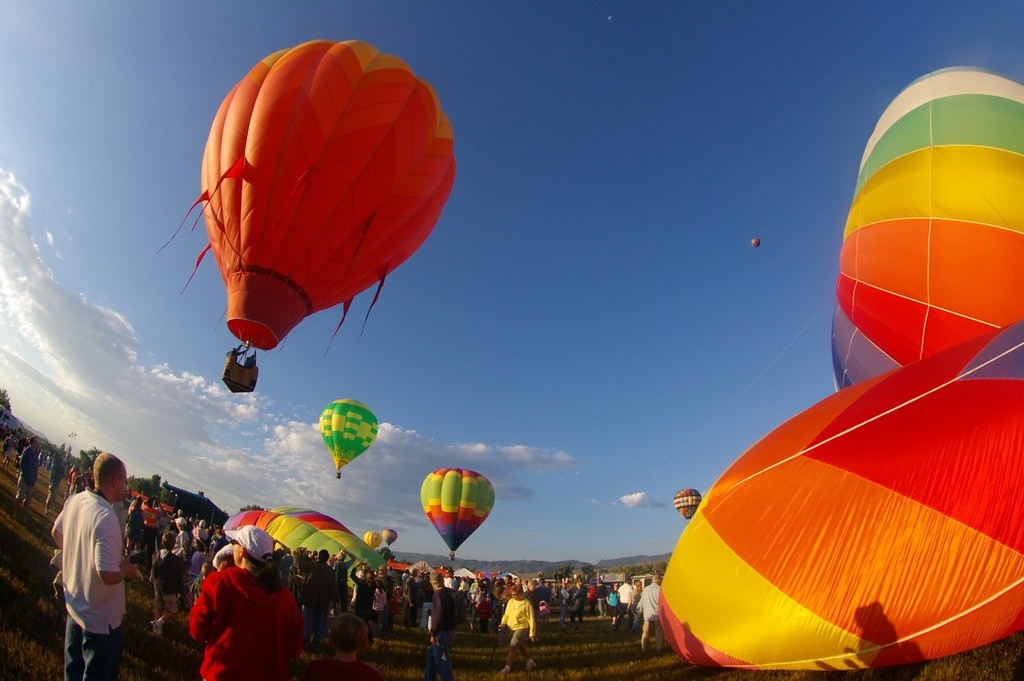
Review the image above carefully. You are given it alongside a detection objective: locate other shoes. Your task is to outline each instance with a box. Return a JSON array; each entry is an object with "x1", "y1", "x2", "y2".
[{"x1": 470, "y1": 623, "x2": 474, "y2": 631}]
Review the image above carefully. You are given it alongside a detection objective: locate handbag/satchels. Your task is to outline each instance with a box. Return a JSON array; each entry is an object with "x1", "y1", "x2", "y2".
[
  {"x1": 539, "y1": 600, "x2": 550, "y2": 616},
  {"x1": 3, "y1": 441, "x2": 9, "y2": 449},
  {"x1": 163, "y1": 578, "x2": 180, "y2": 594}
]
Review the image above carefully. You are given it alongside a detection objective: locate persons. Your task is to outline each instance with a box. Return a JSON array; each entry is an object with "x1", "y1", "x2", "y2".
[
  {"x1": 230, "y1": 344, "x2": 247, "y2": 362},
  {"x1": 52, "y1": 452, "x2": 137, "y2": 681},
  {"x1": 299, "y1": 613, "x2": 384, "y2": 681},
  {"x1": 498, "y1": 584, "x2": 536, "y2": 674},
  {"x1": 0, "y1": 420, "x2": 666, "y2": 657},
  {"x1": 422, "y1": 573, "x2": 457, "y2": 681},
  {"x1": 189, "y1": 525, "x2": 304, "y2": 681}
]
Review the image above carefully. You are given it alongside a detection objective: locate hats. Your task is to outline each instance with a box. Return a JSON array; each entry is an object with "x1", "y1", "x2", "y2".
[
  {"x1": 430, "y1": 571, "x2": 444, "y2": 582},
  {"x1": 53, "y1": 452, "x2": 61, "y2": 457},
  {"x1": 175, "y1": 517, "x2": 186, "y2": 526},
  {"x1": 178, "y1": 509, "x2": 183, "y2": 514},
  {"x1": 598, "y1": 580, "x2": 603, "y2": 584},
  {"x1": 225, "y1": 524, "x2": 274, "y2": 563},
  {"x1": 199, "y1": 519, "x2": 206, "y2": 527},
  {"x1": 196, "y1": 536, "x2": 206, "y2": 546}
]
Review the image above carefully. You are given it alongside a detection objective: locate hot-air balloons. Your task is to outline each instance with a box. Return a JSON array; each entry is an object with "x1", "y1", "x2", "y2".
[
  {"x1": 420, "y1": 468, "x2": 495, "y2": 560},
  {"x1": 751, "y1": 238, "x2": 760, "y2": 249},
  {"x1": 363, "y1": 531, "x2": 382, "y2": 550},
  {"x1": 155, "y1": 39, "x2": 455, "y2": 394},
  {"x1": 831, "y1": 65, "x2": 1023, "y2": 390},
  {"x1": 382, "y1": 529, "x2": 398, "y2": 548},
  {"x1": 319, "y1": 398, "x2": 379, "y2": 479},
  {"x1": 222, "y1": 506, "x2": 399, "y2": 600},
  {"x1": 657, "y1": 321, "x2": 1024, "y2": 669},
  {"x1": 672, "y1": 488, "x2": 701, "y2": 524}
]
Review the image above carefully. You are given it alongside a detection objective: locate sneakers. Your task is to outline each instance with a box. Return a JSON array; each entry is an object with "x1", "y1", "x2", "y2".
[
  {"x1": 526, "y1": 661, "x2": 537, "y2": 670},
  {"x1": 501, "y1": 668, "x2": 510, "y2": 673},
  {"x1": 150, "y1": 621, "x2": 159, "y2": 635}
]
